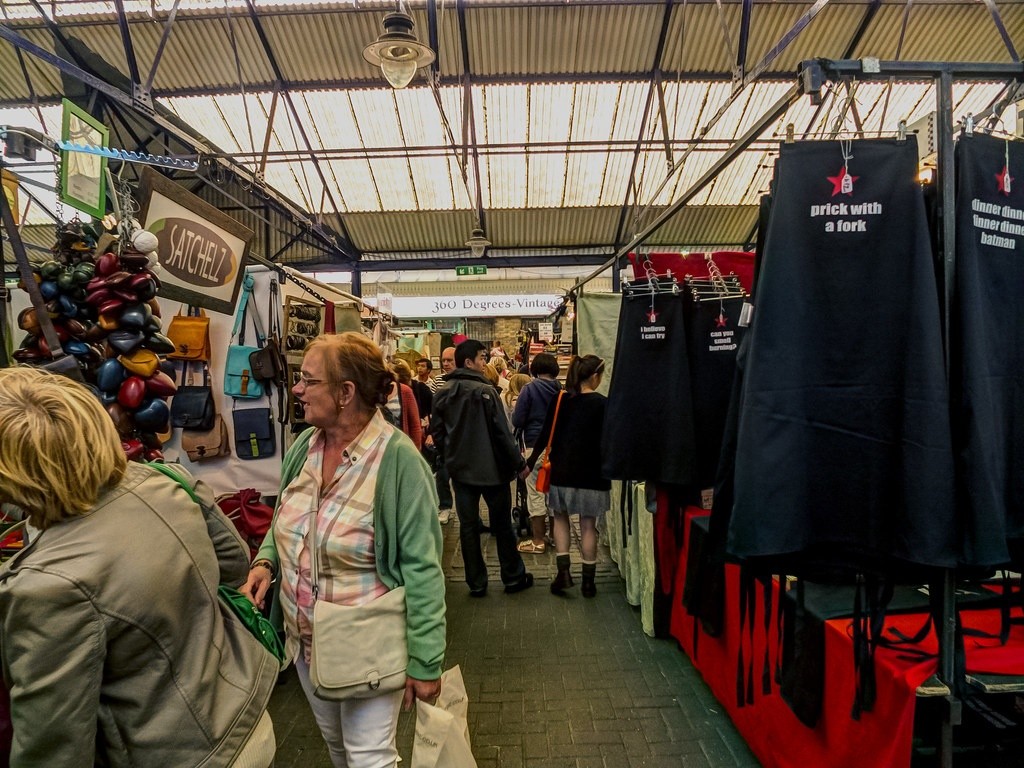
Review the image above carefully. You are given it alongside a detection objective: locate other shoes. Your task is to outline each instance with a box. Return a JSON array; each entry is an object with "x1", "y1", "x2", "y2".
[
  {"x1": 478, "y1": 526, "x2": 490, "y2": 534},
  {"x1": 504, "y1": 573, "x2": 533, "y2": 594},
  {"x1": 469, "y1": 586, "x2": 486, "y2": 596}
]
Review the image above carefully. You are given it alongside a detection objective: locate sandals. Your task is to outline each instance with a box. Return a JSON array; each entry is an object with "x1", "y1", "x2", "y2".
[
  {"x1": 515, "y1": 539, "x2": 546, "y2": 554},
  {"x1": 543, "y1": 532, "x2": 556, "y2": 547}
]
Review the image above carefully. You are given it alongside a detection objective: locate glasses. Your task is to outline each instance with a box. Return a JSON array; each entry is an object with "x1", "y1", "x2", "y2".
[
  {"x1": 299, "y1": 372, "x2": 338, "y2": 386},
  {"x1": 595, "y1": 358, "x2": 606, "y2": 373}
]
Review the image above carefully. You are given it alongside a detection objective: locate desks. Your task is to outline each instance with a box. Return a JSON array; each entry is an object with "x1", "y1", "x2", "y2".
[
  {"x1": 603, "y1": 480, "x2": 654, "y2": 639},
  {"x1": 653, "y1": 493, "x2": 1024, "y2": 768}
]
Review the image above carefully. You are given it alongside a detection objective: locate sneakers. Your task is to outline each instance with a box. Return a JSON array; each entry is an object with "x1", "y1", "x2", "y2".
[{"x1": 437, "y1": 509, "x2": 456, "y2": 525}]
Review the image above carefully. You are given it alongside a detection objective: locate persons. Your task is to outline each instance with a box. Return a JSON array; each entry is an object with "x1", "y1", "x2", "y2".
[
  {"x1": 485, "y1": 340, "x2": 532, "y2": 436},
  {"x1": 239, "y1": 331, "x2": 446, "y2": 767},
  {"x1": 386, "y1": 359, "x2": 434, "y2": 472},
  {"x1": 426, "y1": 347, "x2": 457, "y2": 524},
  {"x1": 523, "y1": 353, "x2": 612, "y2": 597},
  {"x1": 431, "y1": 340, "x2": 533, "y2": 597},
  {"x1": 0, "y1": 365, "x2": 278, "y2": 767}
]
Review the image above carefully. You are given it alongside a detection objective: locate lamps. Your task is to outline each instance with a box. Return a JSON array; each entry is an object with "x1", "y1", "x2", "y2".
[
  {"x1": 464, "y1": 218, "x2": 492, "y2": 257},
  {"x1": 360, "y1": 0, "x2": 437, "y2": 91}
]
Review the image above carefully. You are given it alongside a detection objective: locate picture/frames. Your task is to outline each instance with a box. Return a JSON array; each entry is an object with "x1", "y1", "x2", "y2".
[
  {"x1": 59, "y1": 98, "x2": 110, "y2": 222},
  {"x1": 129, "y1": 165, "x2": 255, "y2": 316}
]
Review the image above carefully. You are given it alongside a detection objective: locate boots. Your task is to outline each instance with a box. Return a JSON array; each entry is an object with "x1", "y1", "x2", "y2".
[
  {"x1": 581, "y1": 560, "x2": 596, "y2": 597},
  {"x1": 551, "y1": 553, "x2": 575, "y2": 591}
]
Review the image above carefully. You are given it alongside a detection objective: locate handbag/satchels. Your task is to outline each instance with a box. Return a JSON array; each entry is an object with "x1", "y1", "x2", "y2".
[
  {"x1": 180, "y1": 413, "x2": 231, "y2": 462},
  {"x1": 308, "y1": 586, "x2": 412, "y2": 701},
  {"x1": 204, "y1": 488, "x2": 280, "y2": 542},
  {"x1": 164, "y1": 300, "x2": 211, "y2": 363},
  {"x1": 536, "y1": 461, "x2": 552, "y2": 494},
  {"x1": 145, "y1": 461, "x2": 288, "y2": 666},
  {"x1": 169, "y1": 362, "x2": 215, "y2": 432},
  {"x1": 410, "y1": 665, "x2": 477, "y2": 768},
  {"x1": 223, "y1": 275, "x2": 292, "y2": 460}
]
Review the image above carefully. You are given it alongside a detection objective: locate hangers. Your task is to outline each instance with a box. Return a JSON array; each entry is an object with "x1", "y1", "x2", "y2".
[
  {"x1": 619, "y1": 260, "x2": 683, "y2": 300},
  {"x1": 755, "y1": 96, "x2": 919, "y2": 194},
  {"x1": 684, "y1": 259, "x2": 751, "y2": 303},
  {"x1": 956, "y1": 98, "x2": 1024, "y2": 143}
]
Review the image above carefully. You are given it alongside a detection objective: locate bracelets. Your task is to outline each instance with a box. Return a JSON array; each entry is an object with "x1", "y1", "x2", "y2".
[{"x1": 251, "y1": 561, "x2": 276, "y2": 581}]
